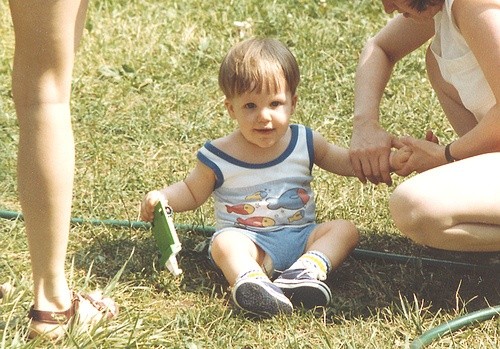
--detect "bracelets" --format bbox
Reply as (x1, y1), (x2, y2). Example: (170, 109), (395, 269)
(445, 142), (460, 163)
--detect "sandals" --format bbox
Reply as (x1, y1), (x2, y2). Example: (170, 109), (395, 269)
(25, 291), (119, 340)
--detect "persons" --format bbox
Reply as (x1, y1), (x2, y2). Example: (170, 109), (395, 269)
(140, 37), (440, 319)
(349, 0), (499, 312)
(0, 0), (120, 342)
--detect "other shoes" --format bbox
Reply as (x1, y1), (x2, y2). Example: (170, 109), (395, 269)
(273, 267), (332, 307)
(232, 278), (293, 317)
